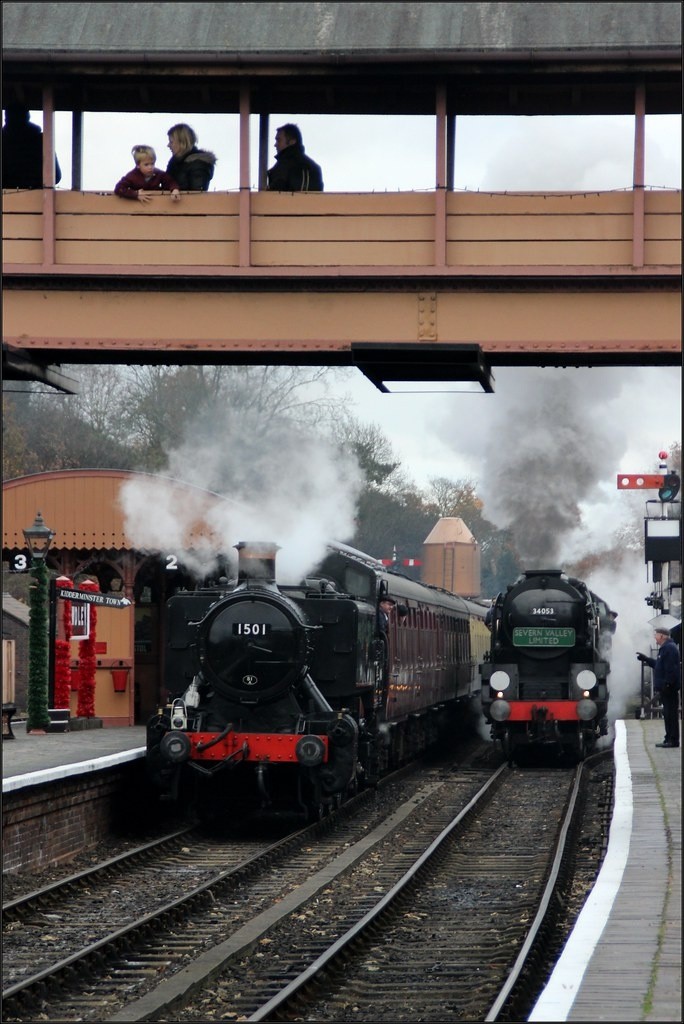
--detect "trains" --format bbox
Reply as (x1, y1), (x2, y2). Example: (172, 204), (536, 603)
(480, 569), (619, 768)
(134, 535), (492, 839)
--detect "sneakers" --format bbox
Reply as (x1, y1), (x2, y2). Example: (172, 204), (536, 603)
(654, 741), (680, 748)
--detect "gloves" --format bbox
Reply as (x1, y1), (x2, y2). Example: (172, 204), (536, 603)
(663, 683), (672, 697)
(635, 652), (647, 662)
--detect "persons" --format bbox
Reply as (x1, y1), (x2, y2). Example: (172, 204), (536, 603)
(636, 628), (682, 749)
(113, 146), (180, 204)
(1, 105), (61, 189)
(266, 123), (324, 192)
(377, 596), (396, 662)
(164, 123), (218, 192)
(396, 604), (409, 628)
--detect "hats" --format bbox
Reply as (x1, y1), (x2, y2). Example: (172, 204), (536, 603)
(654, 627), (670, 636)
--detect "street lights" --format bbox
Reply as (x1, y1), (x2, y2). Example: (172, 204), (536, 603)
(23, 511), (56, 735)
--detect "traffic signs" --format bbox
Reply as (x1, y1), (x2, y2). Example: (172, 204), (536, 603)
(59, 588), (132, 609)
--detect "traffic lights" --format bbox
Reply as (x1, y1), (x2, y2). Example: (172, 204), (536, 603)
(658, 470), (681, 504)
